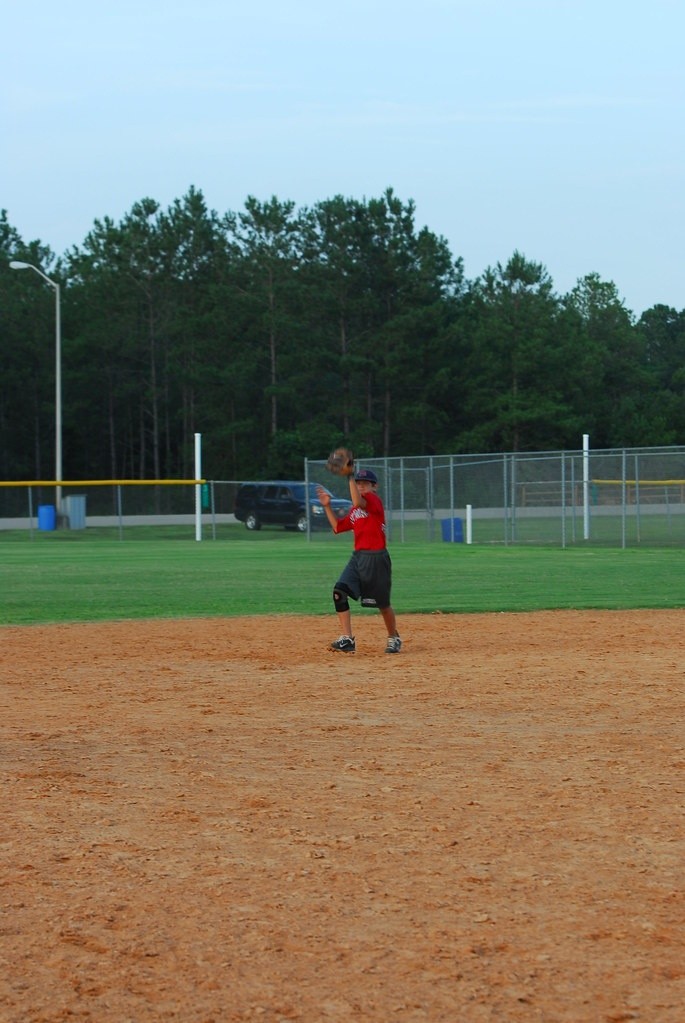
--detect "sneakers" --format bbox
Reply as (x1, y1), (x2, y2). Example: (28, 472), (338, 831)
(385, 630), (400, 652)
(326, 636), (356, 654)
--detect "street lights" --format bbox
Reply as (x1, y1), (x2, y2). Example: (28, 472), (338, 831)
(9, 261), (63, 519)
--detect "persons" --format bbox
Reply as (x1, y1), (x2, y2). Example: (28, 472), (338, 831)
(314, 446), (405, 654)
(281, 490), (291, 498)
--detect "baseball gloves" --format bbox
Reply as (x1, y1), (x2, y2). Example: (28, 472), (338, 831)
(325, 446), (355, 478)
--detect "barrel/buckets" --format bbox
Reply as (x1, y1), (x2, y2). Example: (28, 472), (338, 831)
(38, 505), (55, 530)
(441, 517), (463, 542)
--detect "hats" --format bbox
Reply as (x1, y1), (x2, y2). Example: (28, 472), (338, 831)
(353, 470), (377, 484)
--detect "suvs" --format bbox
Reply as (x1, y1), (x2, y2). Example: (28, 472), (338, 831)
(233, 481), (353, 533)
